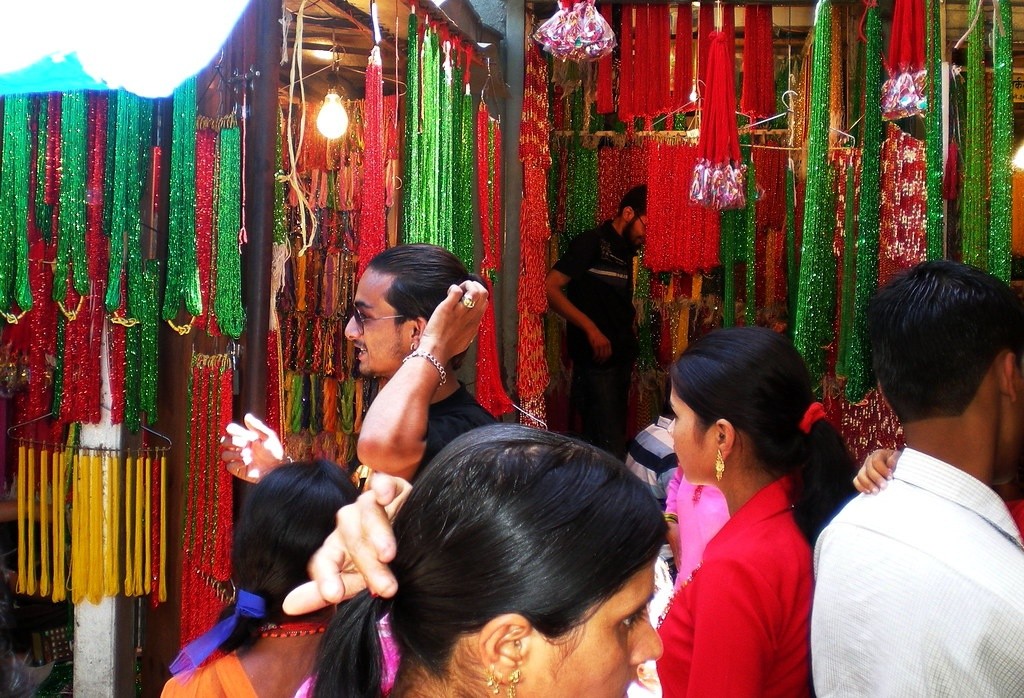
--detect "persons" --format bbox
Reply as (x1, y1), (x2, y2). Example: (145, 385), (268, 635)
(812, 260), (1024, 698)
(664, 463), (730, 593)
(623, 378), (679, 511)
(546, 184), (648, 464)
(655, 327), (860, 698)
(161, 459), (362, 698)
(853, 448), (1024, 537)
(219, 243), (498, 484)
(283, 423), (664, 698)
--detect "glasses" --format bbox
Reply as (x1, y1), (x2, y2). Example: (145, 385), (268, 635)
(638, 216), (646, 233)
(349, 303), (404, 335)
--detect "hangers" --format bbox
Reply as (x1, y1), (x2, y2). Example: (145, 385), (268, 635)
(511, 404), (547, 432)
(7, 397), (173, 454)
(190, 329), (236, 358)
(647, 79), (752, 132)
(480, 56), (504, 123)
(196, 49), (239, 117)
(278, 43), (407, 102)
(194, 565), (236, 605)
(739, 91), (856, 149)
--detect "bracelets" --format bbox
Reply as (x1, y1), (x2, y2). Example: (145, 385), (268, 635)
(286, 456), (292, 465)
(403, 352), (446, 388)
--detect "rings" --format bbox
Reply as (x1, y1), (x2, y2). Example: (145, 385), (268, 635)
(462, 295), (474, 308)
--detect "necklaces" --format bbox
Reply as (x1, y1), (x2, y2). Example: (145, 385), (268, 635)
(657, 563), (700, 627)
(263, 627), (325, 639)
